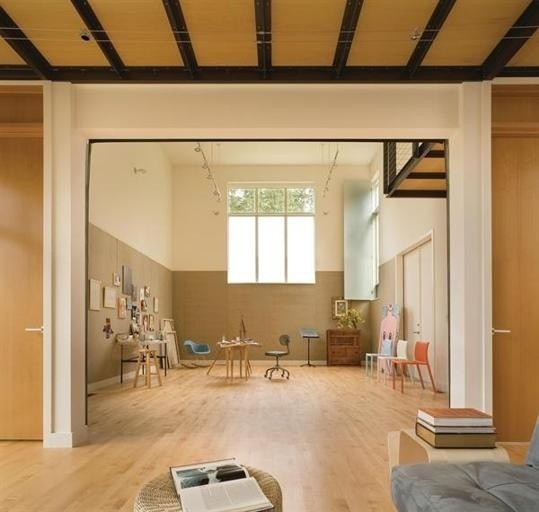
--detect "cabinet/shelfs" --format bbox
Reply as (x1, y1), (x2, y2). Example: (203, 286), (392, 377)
(326, 328), (364, 367)
(383, 140), (447, 200)
(397, 428), (511, 465)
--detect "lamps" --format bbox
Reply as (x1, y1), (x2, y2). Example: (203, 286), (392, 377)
(317, 142), (340, 198)
(195, 142), (224, 204)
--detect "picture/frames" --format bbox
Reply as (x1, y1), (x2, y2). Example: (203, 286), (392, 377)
(89, 266), (160, 332)
(164, 331), (180, 369)
(330, 295), (352, 321)
(161, 319), (175, 368)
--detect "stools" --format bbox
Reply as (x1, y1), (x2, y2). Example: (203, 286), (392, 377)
(133, 349), (163, 389)
(223, 345), (244, 380)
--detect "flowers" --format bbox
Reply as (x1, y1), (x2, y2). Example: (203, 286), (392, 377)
(336, 308), (366, 328)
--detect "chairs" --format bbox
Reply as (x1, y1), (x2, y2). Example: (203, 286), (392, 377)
(181, 339), (214, 372)
(263, 335), (292, 380)
(376, 340), (413, 386)
(391, 413), (539, 512)
(392, 341), (438, 394)
(366, 338), (394, 378)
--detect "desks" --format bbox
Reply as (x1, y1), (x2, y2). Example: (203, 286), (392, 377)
(118, 339), (169, 385)
(299, 329), (320, 367)
(206, 342), (259, 376)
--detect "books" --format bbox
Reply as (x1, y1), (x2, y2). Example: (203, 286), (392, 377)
(416, 407), (499, 449)
(169, 457), (275, 512)
